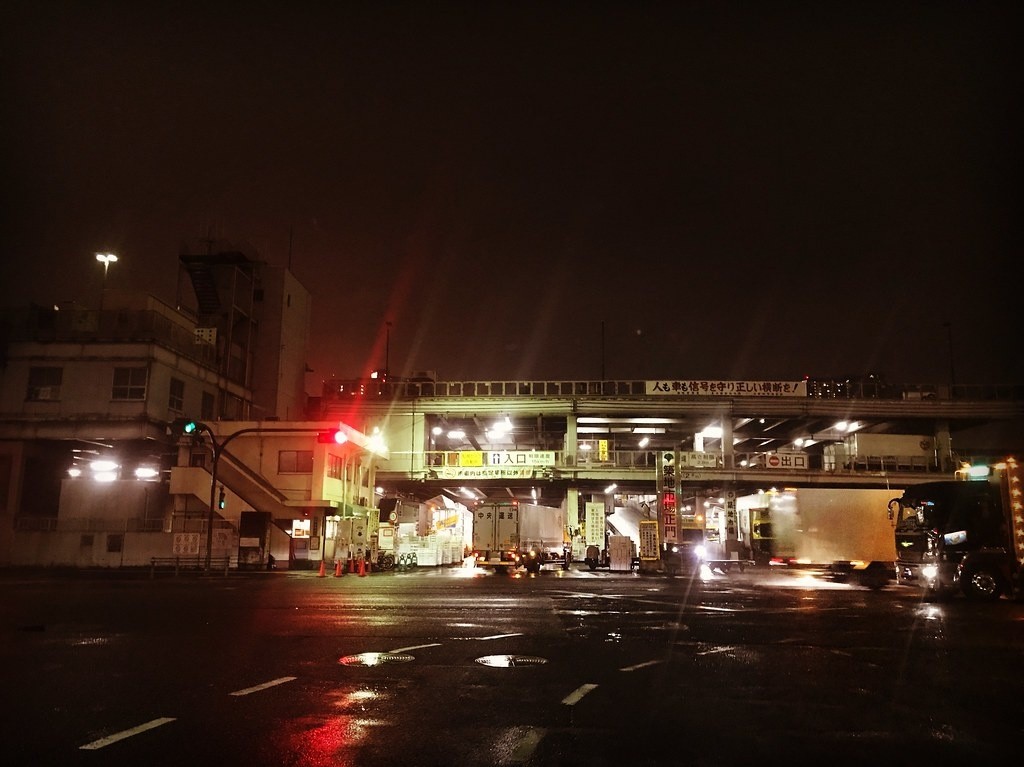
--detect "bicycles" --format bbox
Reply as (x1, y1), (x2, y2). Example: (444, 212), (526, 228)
(378, 550), (395, 572)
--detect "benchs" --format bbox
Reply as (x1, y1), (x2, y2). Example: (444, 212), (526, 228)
(149, 554), (230, 581)
(854, 453), (930, 473)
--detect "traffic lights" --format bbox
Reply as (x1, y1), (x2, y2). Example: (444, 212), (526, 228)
(219, 487), (226, 509)
(174, 421), (196, 432)
(316, 432), (347, 444)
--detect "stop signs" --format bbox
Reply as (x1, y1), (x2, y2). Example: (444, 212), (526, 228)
(769, 456), (780, 467)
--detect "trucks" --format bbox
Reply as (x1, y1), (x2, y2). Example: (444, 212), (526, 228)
(471, 498), (572, 574)
(886, 467), (1024, 601)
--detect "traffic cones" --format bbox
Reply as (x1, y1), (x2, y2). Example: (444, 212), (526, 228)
(348, 558), (356, 574)
(333, 559), (344, 577)
(358, 558), (372, 577)
(316, 560), (328, 578)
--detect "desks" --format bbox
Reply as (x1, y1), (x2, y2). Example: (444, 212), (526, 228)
(705, 559), (748, 574)
